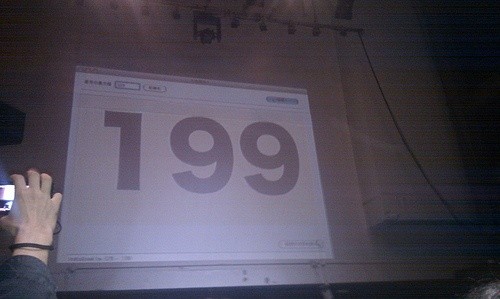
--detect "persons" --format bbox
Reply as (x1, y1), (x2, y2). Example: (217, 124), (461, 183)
(1, 169), (62, 299)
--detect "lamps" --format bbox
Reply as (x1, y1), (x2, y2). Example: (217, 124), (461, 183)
(69, 0), (365, 48)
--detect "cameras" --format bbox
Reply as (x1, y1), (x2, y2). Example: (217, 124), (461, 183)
(0, 183), (54, 216)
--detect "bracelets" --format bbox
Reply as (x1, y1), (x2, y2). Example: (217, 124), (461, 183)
(10, 243), (54, 250)
